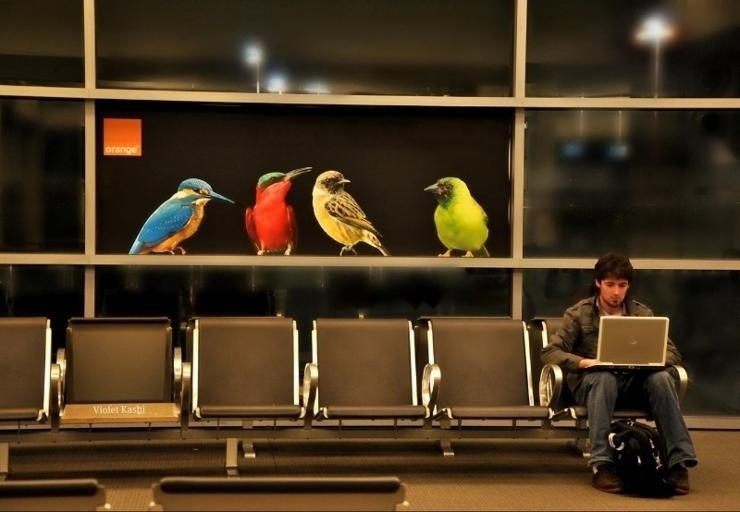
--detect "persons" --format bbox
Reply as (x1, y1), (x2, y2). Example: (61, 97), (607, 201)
(539, 252), (698, 496)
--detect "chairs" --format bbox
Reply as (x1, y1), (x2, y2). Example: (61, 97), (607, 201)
(310, 316), (441, 430)
(537, 315), (688, 425)
(422, 315), (562, 424)
(192, 315), (317, 431)
(49, 317), (187, 435)
(2, 316), (56, 434)
(148, 476), (411, 512)
(0, 474), (108, 511)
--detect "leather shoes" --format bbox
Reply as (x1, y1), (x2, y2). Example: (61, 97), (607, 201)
(591, 466), (621, 493)
(674, 470), (690, 495)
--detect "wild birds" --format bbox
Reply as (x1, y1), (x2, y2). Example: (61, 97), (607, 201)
(128, 176), (236, 256)
(423, 176), (494, 258)
(311, 170), (400, 257)
(243, 164), (314, 255)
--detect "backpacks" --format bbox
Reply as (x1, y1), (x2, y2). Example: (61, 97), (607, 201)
(608, 416), (669, 499)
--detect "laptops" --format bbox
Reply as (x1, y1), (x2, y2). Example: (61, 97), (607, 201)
(584, 316), (669, 373)
